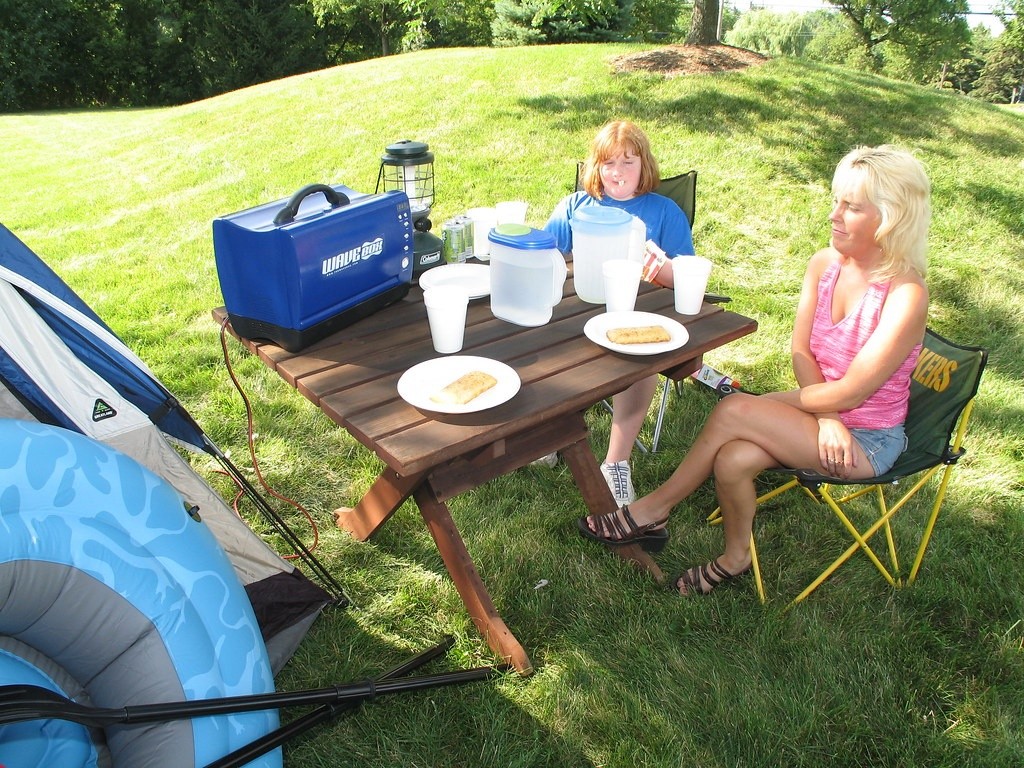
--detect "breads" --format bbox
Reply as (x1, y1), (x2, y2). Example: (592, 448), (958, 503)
(605, 325), (671, 344)
(439, 371), (497, 404)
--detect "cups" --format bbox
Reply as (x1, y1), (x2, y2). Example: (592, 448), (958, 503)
(466, 201), (528, 259)
(422, 289), (469, 354)
(602, 258), (642, 312)
(670, 255), (712, 315)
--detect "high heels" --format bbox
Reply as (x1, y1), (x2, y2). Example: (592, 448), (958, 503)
(578, 504), (669, 553)
(672, 554), (753, 598)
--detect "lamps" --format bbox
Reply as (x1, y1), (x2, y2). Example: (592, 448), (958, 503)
(378, 137), (447, 272)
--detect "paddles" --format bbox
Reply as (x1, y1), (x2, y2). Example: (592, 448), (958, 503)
(0, 663), (508, 733)
(203, 635), (456, 768)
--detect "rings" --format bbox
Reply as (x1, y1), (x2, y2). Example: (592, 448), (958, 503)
(835, 461), (844, 464)
(828, 458), (834, 463)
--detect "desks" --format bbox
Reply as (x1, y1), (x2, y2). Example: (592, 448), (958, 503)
(206, 244), (759, 677)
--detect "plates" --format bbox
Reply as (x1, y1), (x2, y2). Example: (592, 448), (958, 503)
(583, 311), (690, 356)
(418, 262), (490, 301)
(397, 356), (522, 415)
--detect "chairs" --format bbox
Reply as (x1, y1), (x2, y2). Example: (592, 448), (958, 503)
(702, 326), (989, 622)
(570, 157), (711, 456)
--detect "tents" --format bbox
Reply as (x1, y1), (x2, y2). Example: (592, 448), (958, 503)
(0, 222), (349, 690)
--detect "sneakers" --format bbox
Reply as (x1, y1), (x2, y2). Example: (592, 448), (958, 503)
(529, 451), (557, 469)
(600, 459), (634, 509)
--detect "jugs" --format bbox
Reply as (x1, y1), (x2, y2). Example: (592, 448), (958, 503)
(568, 205), (646, 305)
(487, 223), (567, 328)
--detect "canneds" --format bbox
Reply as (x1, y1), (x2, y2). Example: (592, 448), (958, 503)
(440, 215), (475, 264)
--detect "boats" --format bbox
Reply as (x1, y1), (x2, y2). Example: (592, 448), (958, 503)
(0, 419), (282, 768)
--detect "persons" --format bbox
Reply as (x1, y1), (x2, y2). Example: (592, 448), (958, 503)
(578, 144), (931, 596)
(528, 119), (696, 511)
